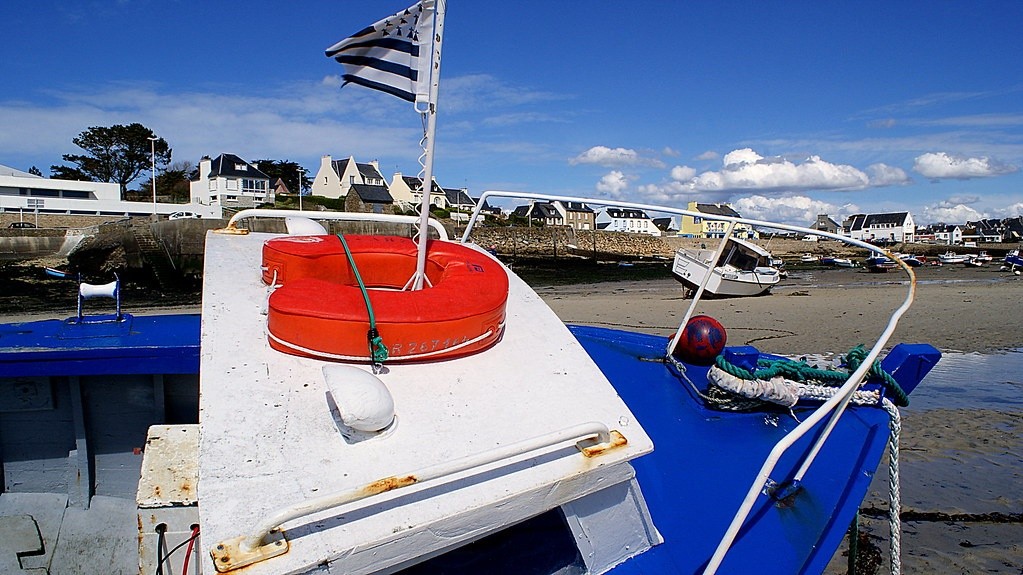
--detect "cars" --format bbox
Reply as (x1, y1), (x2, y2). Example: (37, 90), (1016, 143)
(168, 211), (202, 220)
(8, 222), (43, 228)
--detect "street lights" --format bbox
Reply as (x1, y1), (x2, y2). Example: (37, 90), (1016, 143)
(296, 169), (304, 211)
(458, 189), (465, 227)
(253, 179), (260, 219)
(596, 206), (601, 230)
(147, 137), (159, 214)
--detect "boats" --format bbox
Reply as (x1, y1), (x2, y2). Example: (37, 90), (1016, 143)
(832, 259), (860, 267)
(1005, 249), (1023, 267)
(865, 247), (890, 272)
(671, 233), (781, 298)
(938, 251), (965, 264)
(963, 251), (993, 267)
(44, 265), (78, 280)
(823, 255), (835, 264)
(800, 253), (822, 262)
(0, 1), (942, 575)
(902, 254), (927, 267)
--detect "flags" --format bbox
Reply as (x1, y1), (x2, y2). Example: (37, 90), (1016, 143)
(325, 0), (436, 104)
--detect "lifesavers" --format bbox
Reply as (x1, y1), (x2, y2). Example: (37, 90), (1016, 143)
(261, 234), (509, 364)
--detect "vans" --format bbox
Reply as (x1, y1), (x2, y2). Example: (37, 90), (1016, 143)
(802, 235), (817, 241)
